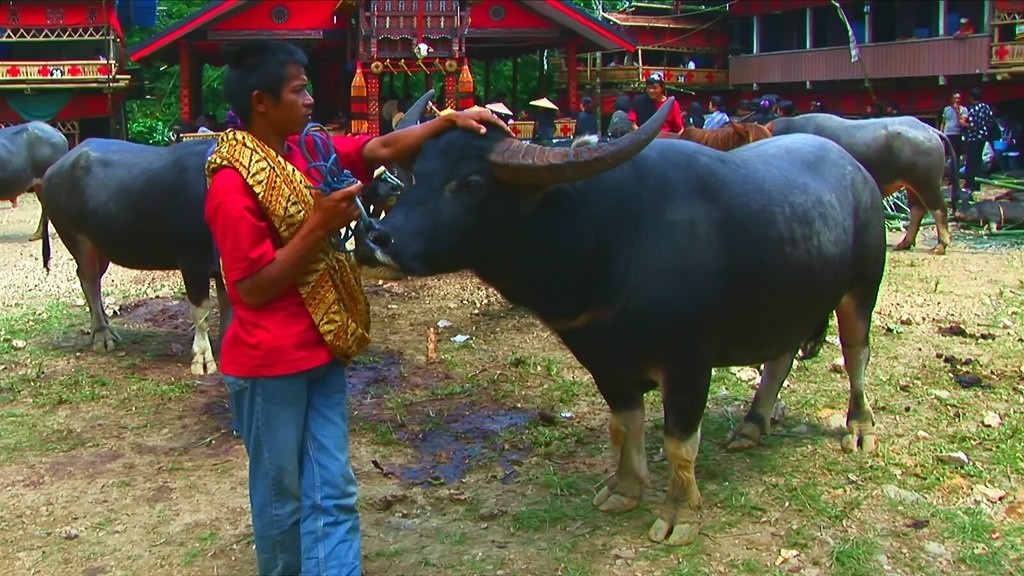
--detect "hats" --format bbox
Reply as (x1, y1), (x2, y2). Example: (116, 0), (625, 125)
(529, 98), (560, 111)
(645, 71), (663, 83)
(485, 102), (513, 116)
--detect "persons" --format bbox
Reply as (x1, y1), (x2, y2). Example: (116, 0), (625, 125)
(678, 53), (696, 83)
(953, 17), (976, 38)
(627, 71), (685, 139)
(487, 94), (905, 148)
(196, 113), (219, 133)
(939, 86), (1023, 195)
(609, 55), (623, 68)
(203, 41), (519, 576)
